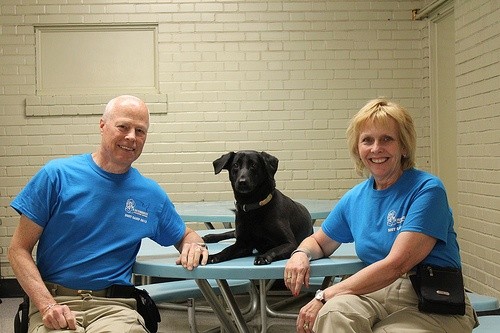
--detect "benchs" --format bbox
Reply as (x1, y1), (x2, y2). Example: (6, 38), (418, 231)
(136, 277), (347, 332)
(466, 292), (500, 333)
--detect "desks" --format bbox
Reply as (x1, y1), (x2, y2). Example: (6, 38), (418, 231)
(133, 228), (366, 333)
(171, 198), (341, 230)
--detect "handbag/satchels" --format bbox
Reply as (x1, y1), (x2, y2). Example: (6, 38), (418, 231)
(409, 264), (467, 317)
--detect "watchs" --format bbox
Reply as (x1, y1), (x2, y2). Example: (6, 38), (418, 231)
(315, 289), (326, 305)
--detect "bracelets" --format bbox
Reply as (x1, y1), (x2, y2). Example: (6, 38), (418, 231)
(290, 248), (312, 261)
(196, 243), (208, 250)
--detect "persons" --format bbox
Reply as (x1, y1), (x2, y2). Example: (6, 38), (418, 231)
(7, 95), (209, 333)
(283, 99), (477, 333)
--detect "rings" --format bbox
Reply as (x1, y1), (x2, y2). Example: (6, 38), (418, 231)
(286, 276), (292, 278)
(303, 324), (308, 328)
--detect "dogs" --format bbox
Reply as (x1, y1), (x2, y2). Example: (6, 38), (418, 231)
(202, 150), (317, 266)
